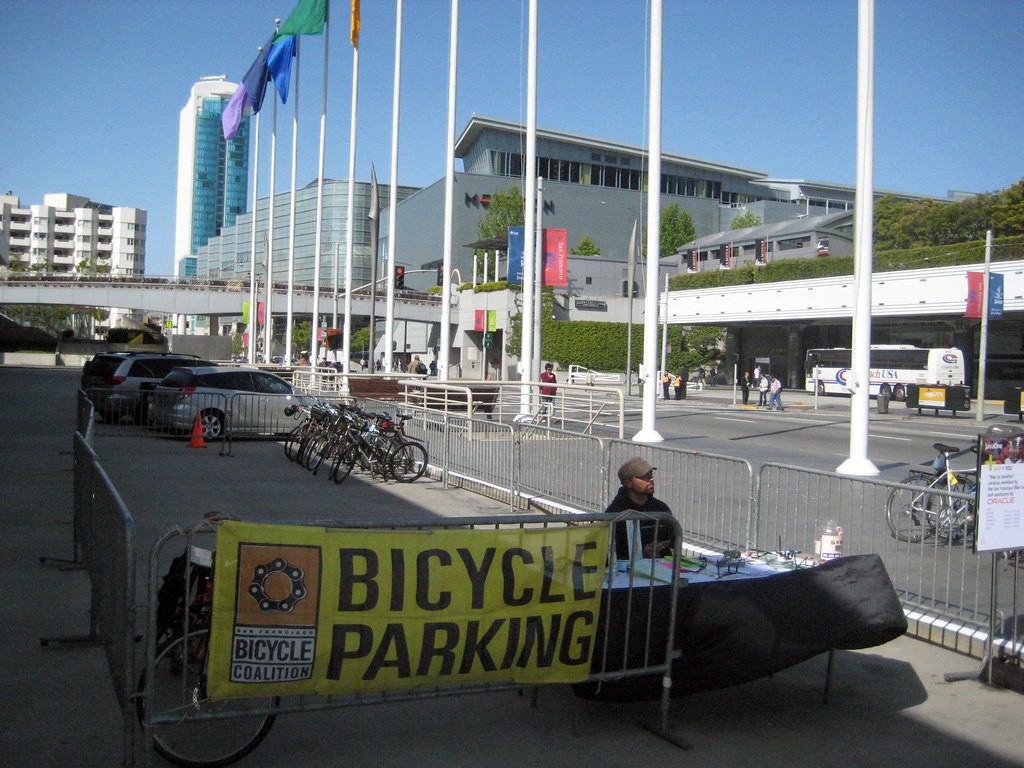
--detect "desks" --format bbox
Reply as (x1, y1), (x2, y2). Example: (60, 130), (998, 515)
(572, 551), (907, 706)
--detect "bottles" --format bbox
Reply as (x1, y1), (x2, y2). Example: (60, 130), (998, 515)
(821, 519), (841, 565)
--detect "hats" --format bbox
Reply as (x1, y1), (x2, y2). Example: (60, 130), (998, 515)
(618, 457), (658, 482)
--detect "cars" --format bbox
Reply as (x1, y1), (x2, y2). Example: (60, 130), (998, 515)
(233, 356), (248, 363)
(272, 356), (283, 363)
(148, 365), (315, 441)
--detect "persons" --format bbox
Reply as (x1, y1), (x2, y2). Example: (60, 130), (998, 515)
(996, 440), (1024, 464)
(698, 364), (718, 387)
(483, 375), (496, 420)
(408, 355), (422, 374)
(539, 363), (560, 424)
(604, 457), (676, 560)
(429, 360), (436, 376)
(663, 370), (671, 400)
(674, 373), (682, 400)
(740, 365), (784, 410)
(360, 357), (404, 372)
(300, 355), (344, 388)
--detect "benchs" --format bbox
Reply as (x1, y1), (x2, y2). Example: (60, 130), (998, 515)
(347, 378), (499, 421)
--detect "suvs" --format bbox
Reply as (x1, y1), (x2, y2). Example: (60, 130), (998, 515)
(80, 351), (220, 425)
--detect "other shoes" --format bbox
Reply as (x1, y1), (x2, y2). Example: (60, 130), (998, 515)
(542, 418), (546, 423)
(553, 419), (559, 424)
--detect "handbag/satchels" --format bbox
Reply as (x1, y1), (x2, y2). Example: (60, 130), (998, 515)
(415, 361), (428, 374)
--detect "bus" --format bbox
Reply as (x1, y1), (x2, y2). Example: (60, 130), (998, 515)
(806, 345), (965, 402)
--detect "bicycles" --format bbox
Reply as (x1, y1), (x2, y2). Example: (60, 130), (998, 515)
(284, 391), (428, 487)
(886, 434), (1024, 559)
(141, 513), (281, 768)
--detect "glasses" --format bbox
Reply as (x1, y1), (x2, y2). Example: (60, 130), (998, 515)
(547, 369), (552, 371)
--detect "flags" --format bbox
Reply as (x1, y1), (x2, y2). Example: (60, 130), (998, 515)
(221, 0), (331, 139)
(351, 0), (361, 48)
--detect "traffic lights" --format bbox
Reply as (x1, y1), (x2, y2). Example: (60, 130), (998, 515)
(395, 266), (405, 289)
(437, 264), (443, 285)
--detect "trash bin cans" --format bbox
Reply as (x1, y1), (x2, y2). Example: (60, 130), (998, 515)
(300, 351), (309, 366)
(878, 393), (890, 413)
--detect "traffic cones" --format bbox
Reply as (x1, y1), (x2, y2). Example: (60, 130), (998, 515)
(188, 411), (207, 448)
